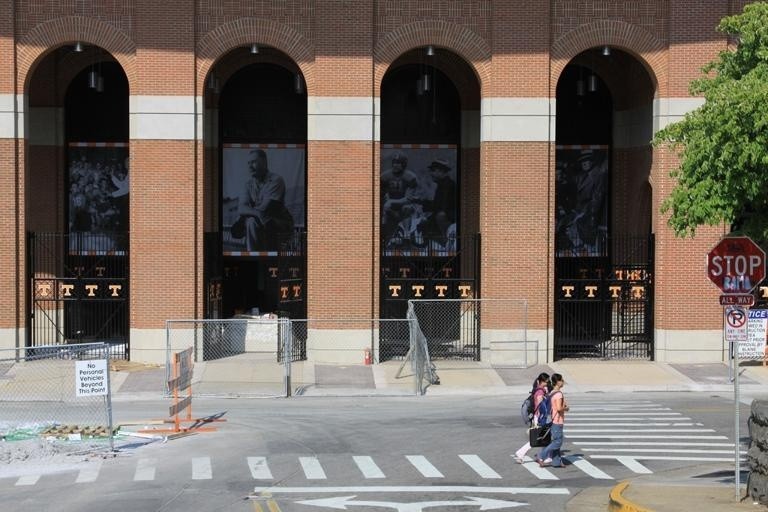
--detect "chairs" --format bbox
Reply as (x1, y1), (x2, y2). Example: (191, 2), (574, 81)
(514, 453), (567, 468)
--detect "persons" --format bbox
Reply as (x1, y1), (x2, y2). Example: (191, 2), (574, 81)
(512, 373), (554, 464)
(68, 152), (129, 252)
(554, 159), (578, 251)
(406, 159), (457, 247)
(534, 373), (569, 469)
(567, 147), (605, 250)
(230, 150), (296, 253)
(380, 151), (420, 251)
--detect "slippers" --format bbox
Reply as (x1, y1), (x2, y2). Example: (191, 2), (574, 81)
(537, 390), (561, 427)
(520, 388), (545, 421)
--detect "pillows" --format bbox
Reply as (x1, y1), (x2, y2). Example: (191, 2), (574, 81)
(391, 150), (408, 167)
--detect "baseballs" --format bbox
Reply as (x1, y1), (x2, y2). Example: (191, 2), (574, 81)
(365, 348), (371, 364)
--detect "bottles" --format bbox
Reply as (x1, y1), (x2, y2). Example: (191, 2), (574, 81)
(427, 159), (452, 171)
(577, 150), (594, 164)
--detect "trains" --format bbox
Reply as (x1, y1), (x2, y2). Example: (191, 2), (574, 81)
(707, 235), (766, 295)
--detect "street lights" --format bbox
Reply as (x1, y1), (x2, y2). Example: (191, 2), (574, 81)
(529, 426), (552, 448)
